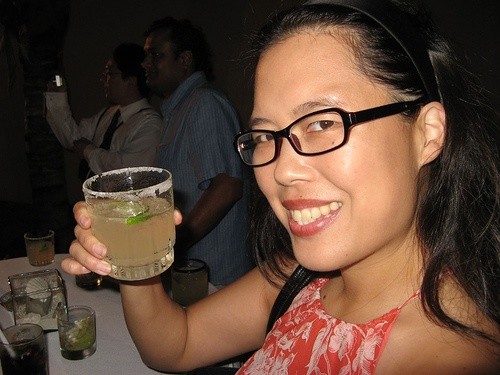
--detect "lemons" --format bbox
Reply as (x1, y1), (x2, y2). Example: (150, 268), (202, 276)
(113, 201), (150, 225)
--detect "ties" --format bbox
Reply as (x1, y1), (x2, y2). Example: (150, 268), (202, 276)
(88, 109), (121, 180)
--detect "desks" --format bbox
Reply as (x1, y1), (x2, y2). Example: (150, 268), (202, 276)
(0, 254), (241, 375)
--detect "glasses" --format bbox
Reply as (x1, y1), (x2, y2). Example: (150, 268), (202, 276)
(232, 97), (422, 168)
(97, 67), (124, 78)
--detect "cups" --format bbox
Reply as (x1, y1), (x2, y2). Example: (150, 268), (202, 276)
(0, 287), (25, 313)
(82, 166), (176, 281)
(0, 323), (46, 375)
(8, 269), (69, 333)
(169, 258), (209, 308)
(24, 229), (54, 267)
(57, 305), (97, 361)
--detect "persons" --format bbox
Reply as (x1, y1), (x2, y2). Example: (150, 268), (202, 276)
(48, 42), (164, 176)
(60, 0), (500, 375)
(141, 18), (249, 290)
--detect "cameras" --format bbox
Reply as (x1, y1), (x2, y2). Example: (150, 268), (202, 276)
(51, 70), (63, 88)
